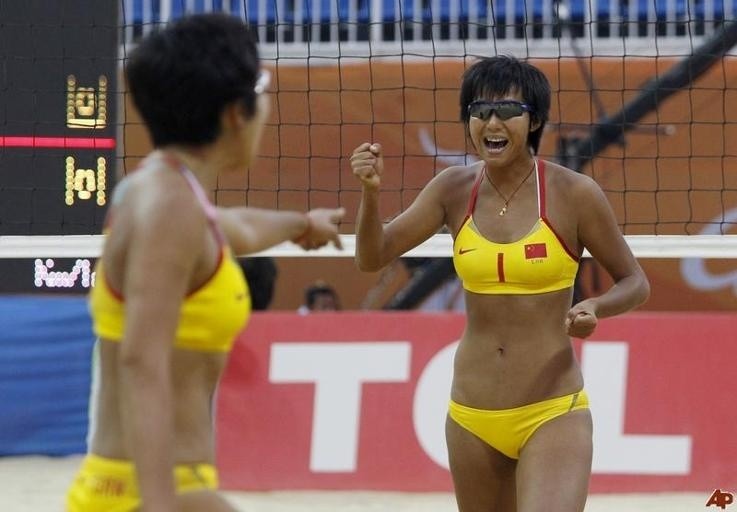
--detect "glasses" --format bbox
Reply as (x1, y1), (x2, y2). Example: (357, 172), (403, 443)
(467, 99), (533, 122)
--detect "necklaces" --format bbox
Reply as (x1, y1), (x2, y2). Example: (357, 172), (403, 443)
(483, 162), (536, 216)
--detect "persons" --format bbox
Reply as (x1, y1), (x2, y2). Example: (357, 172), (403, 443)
(65, 10), (348, 511)
(304, 280), (338, 312)
(349, 52), (651, 510)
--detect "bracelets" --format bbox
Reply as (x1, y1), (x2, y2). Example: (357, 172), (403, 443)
(291, 213), (313, 245)
